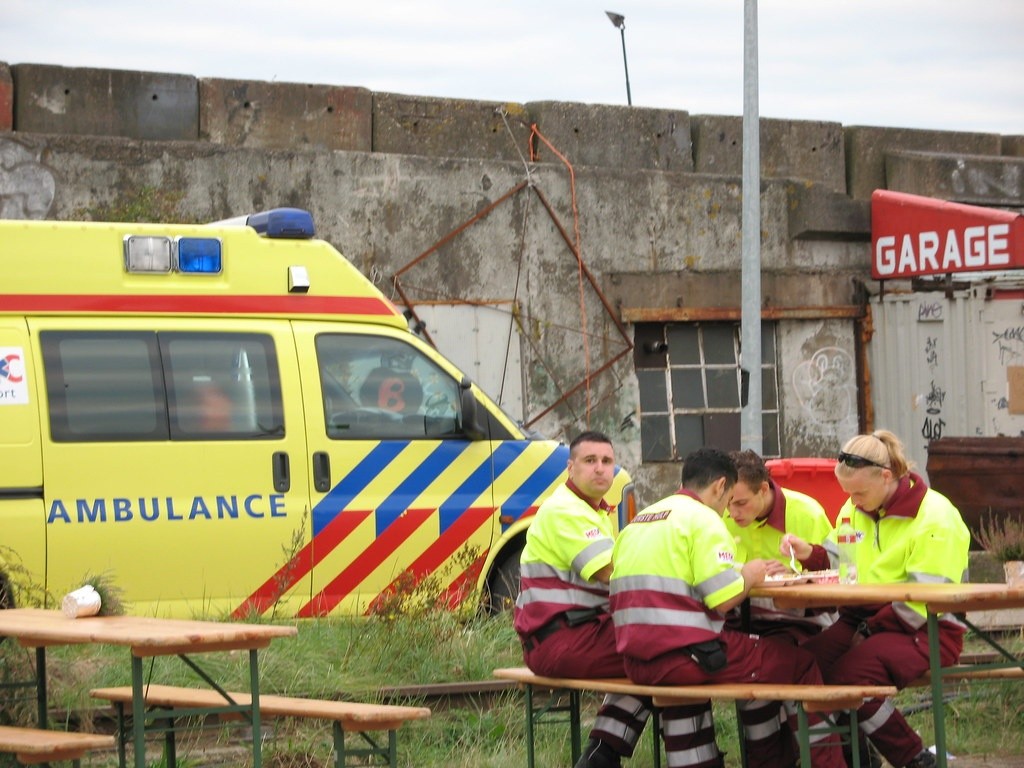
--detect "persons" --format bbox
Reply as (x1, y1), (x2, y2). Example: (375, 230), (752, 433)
(512, 429), (971, 768)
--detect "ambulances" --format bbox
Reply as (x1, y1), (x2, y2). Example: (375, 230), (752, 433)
(0, 207), (647, 620)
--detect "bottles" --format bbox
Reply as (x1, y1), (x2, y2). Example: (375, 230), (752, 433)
(838, 517), (859, 584)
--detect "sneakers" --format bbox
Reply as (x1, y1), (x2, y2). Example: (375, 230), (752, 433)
(574, 740), (623, 768)
(906, 748), (937, 768)
(847, 742), (882, 768)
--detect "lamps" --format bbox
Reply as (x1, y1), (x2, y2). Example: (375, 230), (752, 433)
(606, 12), (631, 106)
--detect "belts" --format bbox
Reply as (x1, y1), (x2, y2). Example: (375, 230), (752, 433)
(525, 618), (561, 653)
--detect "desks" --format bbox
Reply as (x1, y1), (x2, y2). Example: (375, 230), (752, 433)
(0, 609), (297, 768)
(739, 583), (1024, 768)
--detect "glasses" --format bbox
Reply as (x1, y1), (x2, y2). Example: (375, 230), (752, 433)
(838, 451), (890, 470)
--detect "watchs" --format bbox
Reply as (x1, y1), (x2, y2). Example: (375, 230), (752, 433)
(857, 618), (870, 639)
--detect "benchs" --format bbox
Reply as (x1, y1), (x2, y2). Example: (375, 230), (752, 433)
(0, 726), (116, 768)
(909, 667), (1024, 687)
(493, 667), (898, 768)
(90, 687), (431, 768)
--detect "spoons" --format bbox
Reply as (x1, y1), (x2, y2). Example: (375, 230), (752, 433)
(787, 535), (801, 575)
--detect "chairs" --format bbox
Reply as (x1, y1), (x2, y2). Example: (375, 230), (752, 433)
(266, 353), (359, 428)
(184, 383), (233, 434)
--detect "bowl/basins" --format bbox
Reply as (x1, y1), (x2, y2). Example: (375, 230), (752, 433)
(757, 571), (838, 587)
